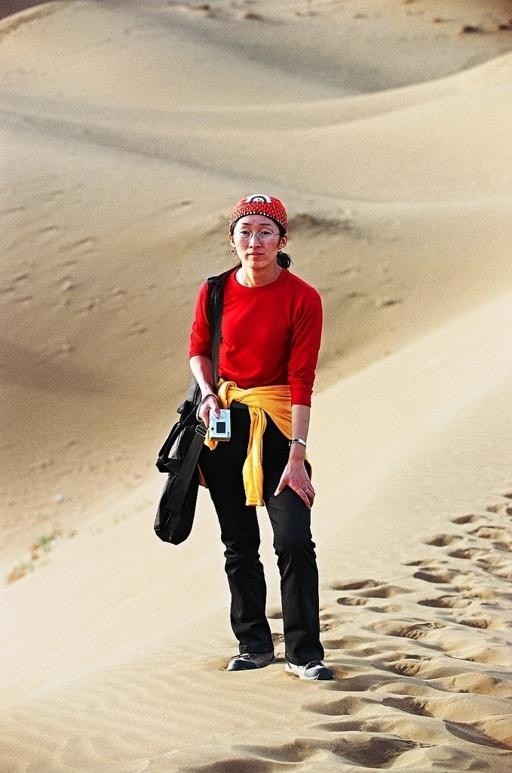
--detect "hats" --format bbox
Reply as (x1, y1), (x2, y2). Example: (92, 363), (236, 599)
(229, 193), (288, 236)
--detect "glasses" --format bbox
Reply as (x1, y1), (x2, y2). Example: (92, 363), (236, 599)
(238, 230), (281, 241)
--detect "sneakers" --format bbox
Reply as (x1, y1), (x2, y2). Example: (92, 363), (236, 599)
(285, 660), (335, 680)
(228, 652), (276, 671)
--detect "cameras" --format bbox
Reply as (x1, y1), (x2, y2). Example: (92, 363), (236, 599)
(209, 409), (231, 441)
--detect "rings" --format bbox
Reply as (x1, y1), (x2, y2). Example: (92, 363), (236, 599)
(305, 489), (309, 493)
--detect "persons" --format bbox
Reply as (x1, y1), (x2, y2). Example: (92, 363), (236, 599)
(187, 193), (339, 684)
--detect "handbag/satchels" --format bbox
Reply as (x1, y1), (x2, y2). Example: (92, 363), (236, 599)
(154, 400), (207, 545)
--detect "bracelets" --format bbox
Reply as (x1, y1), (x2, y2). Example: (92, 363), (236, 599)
(289, 437), (308, 448)
